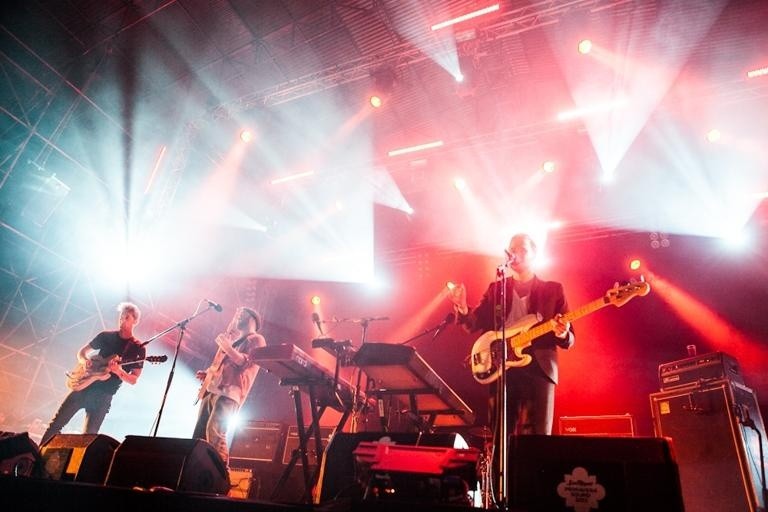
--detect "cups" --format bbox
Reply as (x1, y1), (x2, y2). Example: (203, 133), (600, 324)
(686, 344), (696, 357)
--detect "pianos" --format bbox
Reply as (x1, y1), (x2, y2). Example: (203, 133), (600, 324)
(353, 341), (476, 427)
(249, 344), (375, 413)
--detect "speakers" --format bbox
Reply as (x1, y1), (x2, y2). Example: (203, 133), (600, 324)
(280, 425), (338, 466)
(558, 414), (637, 437)
(103, 435), (232, 495)
(36, 433), (121, 482)
(648, 378), (768, 512)
(318, 431), (484, 507)
(504, 434), (686, 512)
(227, 421), (287, 464)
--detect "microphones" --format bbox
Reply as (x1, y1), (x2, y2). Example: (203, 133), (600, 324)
(205, 298), (223, 312)
(311, 312), (324, 336)
(505, 248), (521, 265)
(432, 312), (456, 338)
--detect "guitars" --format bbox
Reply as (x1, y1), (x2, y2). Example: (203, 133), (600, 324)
(66, 356), (168, 392)
(194, 308), (245, 399)
(471, 276), (649, 384)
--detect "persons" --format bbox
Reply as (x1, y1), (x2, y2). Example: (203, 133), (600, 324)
(190, 306), (267, 470)
(37, 301), (146, 446)
(448, 231), (575, 438)
